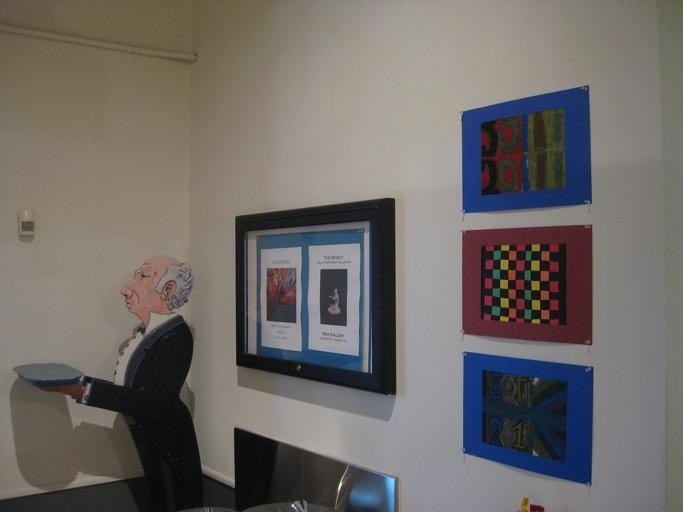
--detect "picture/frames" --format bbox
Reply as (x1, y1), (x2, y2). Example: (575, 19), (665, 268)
(462, 350), (591, 484)
(237, 196), (396, 397)
(461, 226), (593, 345)
(463, 90), (592, 213)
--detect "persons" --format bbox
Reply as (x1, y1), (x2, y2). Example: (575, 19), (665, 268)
(38, 256), (203, 512)
